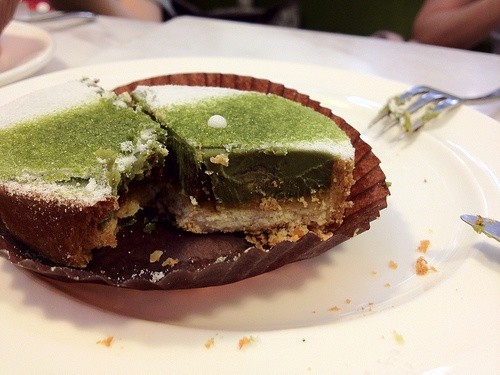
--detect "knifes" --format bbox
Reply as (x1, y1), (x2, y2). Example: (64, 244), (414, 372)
(460, 215), (500, 243)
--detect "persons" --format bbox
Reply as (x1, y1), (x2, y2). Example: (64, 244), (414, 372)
(52, 0), (175, 22)
(413, 0), (500, 51)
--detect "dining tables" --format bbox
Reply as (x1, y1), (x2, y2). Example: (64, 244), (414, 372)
(0, 10), (500, 375)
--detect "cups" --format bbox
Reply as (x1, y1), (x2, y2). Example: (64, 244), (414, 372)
(1, 0), (22, 34)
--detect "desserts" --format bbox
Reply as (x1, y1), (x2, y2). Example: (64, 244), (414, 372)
(130, 83), (357, 236)
(0, 76), (170, 269)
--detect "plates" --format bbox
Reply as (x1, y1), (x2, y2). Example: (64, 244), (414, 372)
(0, 58), (500, 375)
(0, 20), (54, 87)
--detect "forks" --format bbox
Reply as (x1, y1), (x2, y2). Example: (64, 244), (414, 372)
(367, 88), (500, 143)
